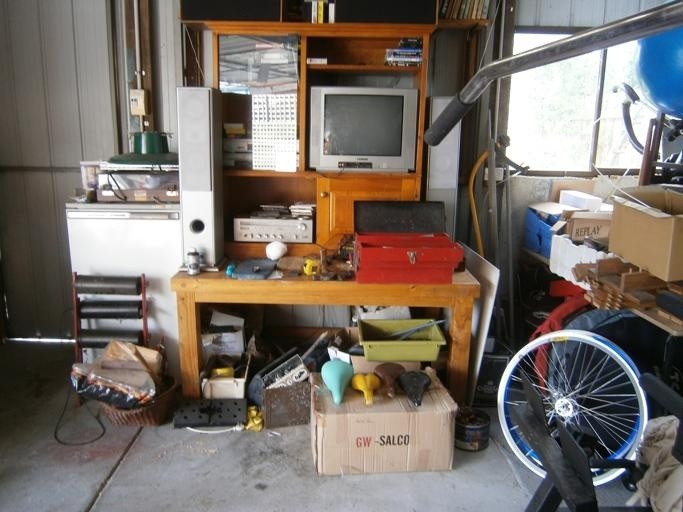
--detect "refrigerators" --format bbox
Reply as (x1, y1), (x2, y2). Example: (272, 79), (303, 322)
(66, 203), (183, 380)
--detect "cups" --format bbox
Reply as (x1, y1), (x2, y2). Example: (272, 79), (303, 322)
(303, 252), (320, 277)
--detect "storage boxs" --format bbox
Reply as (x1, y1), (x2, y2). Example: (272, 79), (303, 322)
(524, 190), (617, 290)
(608, 185), (682, 282)
(310, 368), (457, 471)
(327, 315), (446, 373)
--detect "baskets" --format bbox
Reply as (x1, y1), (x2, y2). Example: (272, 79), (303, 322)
(99, 377), (177, 427)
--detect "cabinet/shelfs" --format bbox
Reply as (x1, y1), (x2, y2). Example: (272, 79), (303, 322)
(171, 22), (435, 255)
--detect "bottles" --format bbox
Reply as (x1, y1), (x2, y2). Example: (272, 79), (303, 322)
(186, 252), (200, 277)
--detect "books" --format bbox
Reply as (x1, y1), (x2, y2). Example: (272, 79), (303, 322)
(384, 47), (422, 67)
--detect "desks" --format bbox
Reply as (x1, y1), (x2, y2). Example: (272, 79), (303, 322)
(171, 260), (483, 398)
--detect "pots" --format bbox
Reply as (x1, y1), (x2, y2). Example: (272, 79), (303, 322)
(128, 131), (175, 154)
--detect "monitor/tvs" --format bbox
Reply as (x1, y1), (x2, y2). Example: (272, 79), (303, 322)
(308, 85), (419, 173)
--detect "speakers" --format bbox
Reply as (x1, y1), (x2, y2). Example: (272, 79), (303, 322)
(176, 87), (228, 273)
(425, 96), (462, 243)
